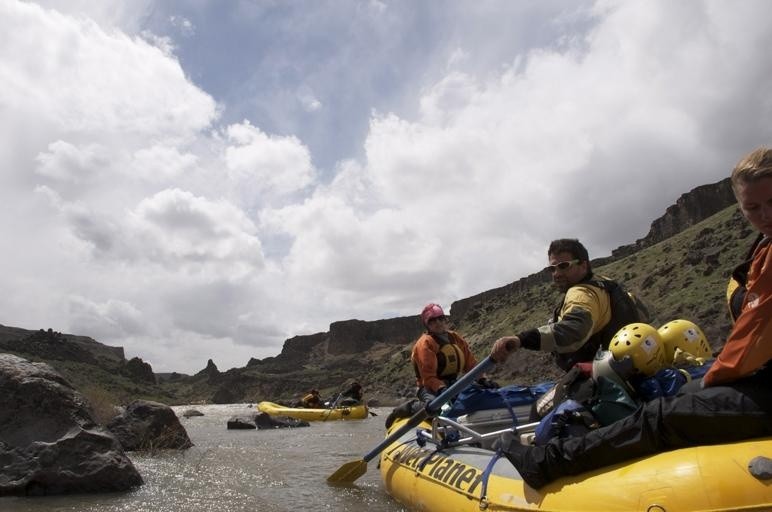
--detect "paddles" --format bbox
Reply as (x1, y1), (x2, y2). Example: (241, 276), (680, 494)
(327, 340), (517, 484)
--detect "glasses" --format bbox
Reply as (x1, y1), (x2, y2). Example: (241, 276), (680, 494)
(428, 315), (446, 325)
(548, 259), (580, 272)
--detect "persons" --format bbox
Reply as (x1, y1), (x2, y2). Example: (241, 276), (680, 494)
(385, 303), (502, 429)
(297, 389), (321, 409)
(489, 236), (649, 378)
(339, 381), (363, 408)
(501, 144), (771, 492)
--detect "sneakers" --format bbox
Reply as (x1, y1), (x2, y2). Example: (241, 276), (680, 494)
(497, 428), (554, 491)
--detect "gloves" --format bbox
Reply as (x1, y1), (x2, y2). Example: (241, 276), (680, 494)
(479, 378), (499, 388)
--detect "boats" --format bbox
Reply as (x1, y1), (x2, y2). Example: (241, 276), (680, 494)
(257, 400), (369, 421)
(380, 411), (772, 512)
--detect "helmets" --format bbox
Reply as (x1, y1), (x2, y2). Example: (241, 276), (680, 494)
(658, 319), (713, 368)
(422, 304), (443, 325)
(592, 350), (638, 398)
(611, 322), (666, 378)
